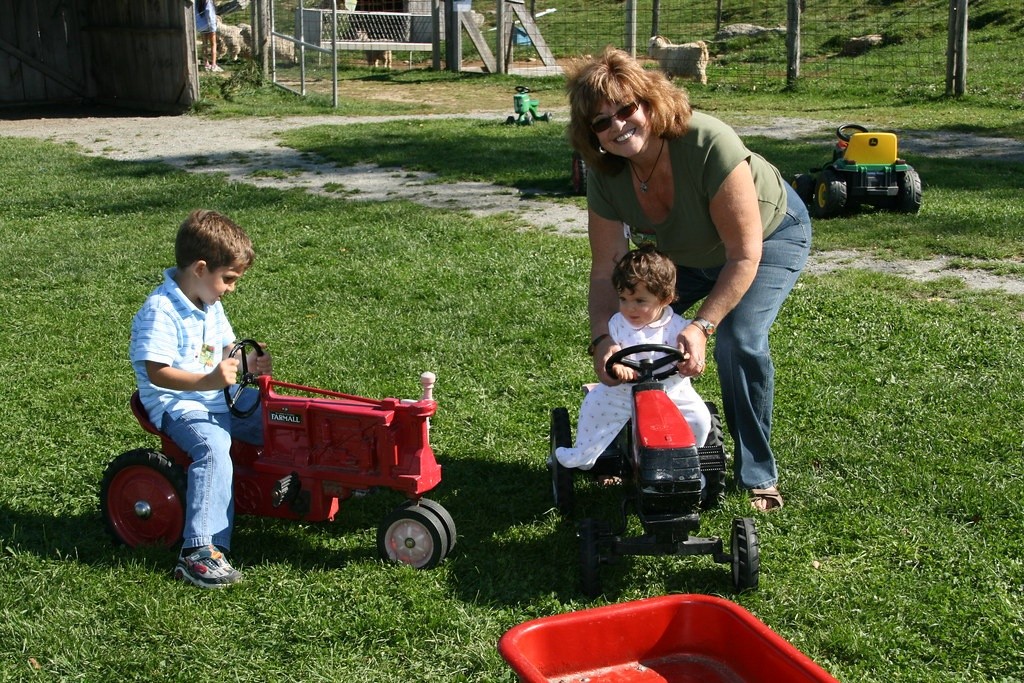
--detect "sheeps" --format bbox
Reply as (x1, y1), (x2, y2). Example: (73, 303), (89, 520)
(215, 15), (298, 65)
(648, 35), (710, 85)
(356, 30), (395, 69)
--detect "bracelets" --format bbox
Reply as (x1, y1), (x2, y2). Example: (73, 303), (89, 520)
(587, 333), (608, 356)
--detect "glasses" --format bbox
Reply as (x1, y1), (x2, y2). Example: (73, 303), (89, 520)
(589, 96), (642, 134)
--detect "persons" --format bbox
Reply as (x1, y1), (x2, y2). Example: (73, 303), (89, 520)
(130, 209), (274, 588)
(561, 48), (813, 513)
(195, 0), (224, 72)
(556, 248), (712, 470)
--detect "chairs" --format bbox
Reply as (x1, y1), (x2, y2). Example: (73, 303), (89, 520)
(130, 388), (173, 444)
(844, 131), (897, 164)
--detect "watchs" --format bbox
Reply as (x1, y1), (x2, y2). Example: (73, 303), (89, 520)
(694, 317), (714, 336)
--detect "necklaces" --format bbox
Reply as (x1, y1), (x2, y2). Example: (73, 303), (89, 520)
(630, 139), (665, 192)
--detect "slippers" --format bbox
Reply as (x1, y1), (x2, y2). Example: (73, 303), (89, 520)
(600, 475), (622, 486)
(745, 486), (783, 511)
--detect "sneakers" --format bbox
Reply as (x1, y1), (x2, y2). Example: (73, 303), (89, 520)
(174, 544), (243, 588)
